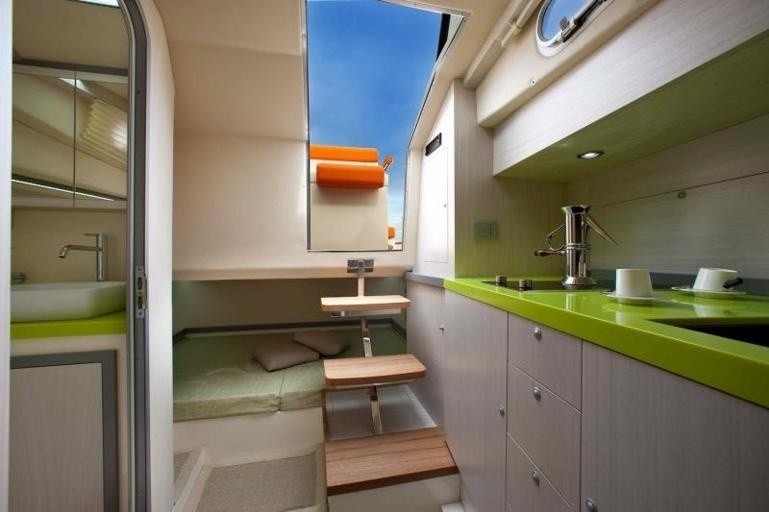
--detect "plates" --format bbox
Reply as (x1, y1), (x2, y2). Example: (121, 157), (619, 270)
(599, 291), (670, 306)
(670, 286), (751, 299)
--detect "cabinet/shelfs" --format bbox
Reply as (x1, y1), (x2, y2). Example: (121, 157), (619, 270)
(404, 270), (768, 511)
(411, 78), (568, 278)
(11, 311), (130, 511)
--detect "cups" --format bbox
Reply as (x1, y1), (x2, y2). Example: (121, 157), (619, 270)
(692, 266), (744, 290)
(613, 266), (657, 297)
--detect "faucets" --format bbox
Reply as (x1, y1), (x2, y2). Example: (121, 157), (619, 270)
(533, 205), (620, 286)
(59, 232), (108, 281)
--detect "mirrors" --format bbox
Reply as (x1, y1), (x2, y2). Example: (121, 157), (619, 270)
(13, 56), (129, 213)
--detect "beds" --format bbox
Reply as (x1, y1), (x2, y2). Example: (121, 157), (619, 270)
(173, 326), (411, 471)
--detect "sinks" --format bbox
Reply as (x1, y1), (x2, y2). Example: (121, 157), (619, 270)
(642, 317), (769, 347)
(11, 281), (127, 323)
